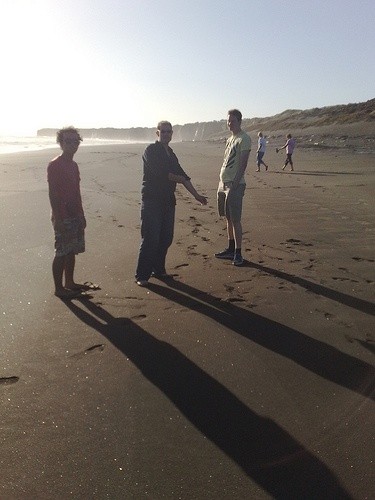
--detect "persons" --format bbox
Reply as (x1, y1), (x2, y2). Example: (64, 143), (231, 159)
(214, 109), (253, 265)
(46, 125), (90, 299)
(256, 132), (268, 172)
(135, 121), (209, 286)
(276, 134), (294, 171)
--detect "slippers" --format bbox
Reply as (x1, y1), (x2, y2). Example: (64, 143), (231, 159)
(77, 289), (90, 296)
(84, 281), (99, 289)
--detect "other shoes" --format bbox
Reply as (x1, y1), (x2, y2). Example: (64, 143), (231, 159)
(152, 269), (165, 276)
(233, 253), (244, 265)
(137, 280), (149, 286)
(214, 248), (234, 259)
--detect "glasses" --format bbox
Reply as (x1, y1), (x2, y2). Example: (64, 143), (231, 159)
(64, 139), (79, 144)
(158, 129), (172, 133)
